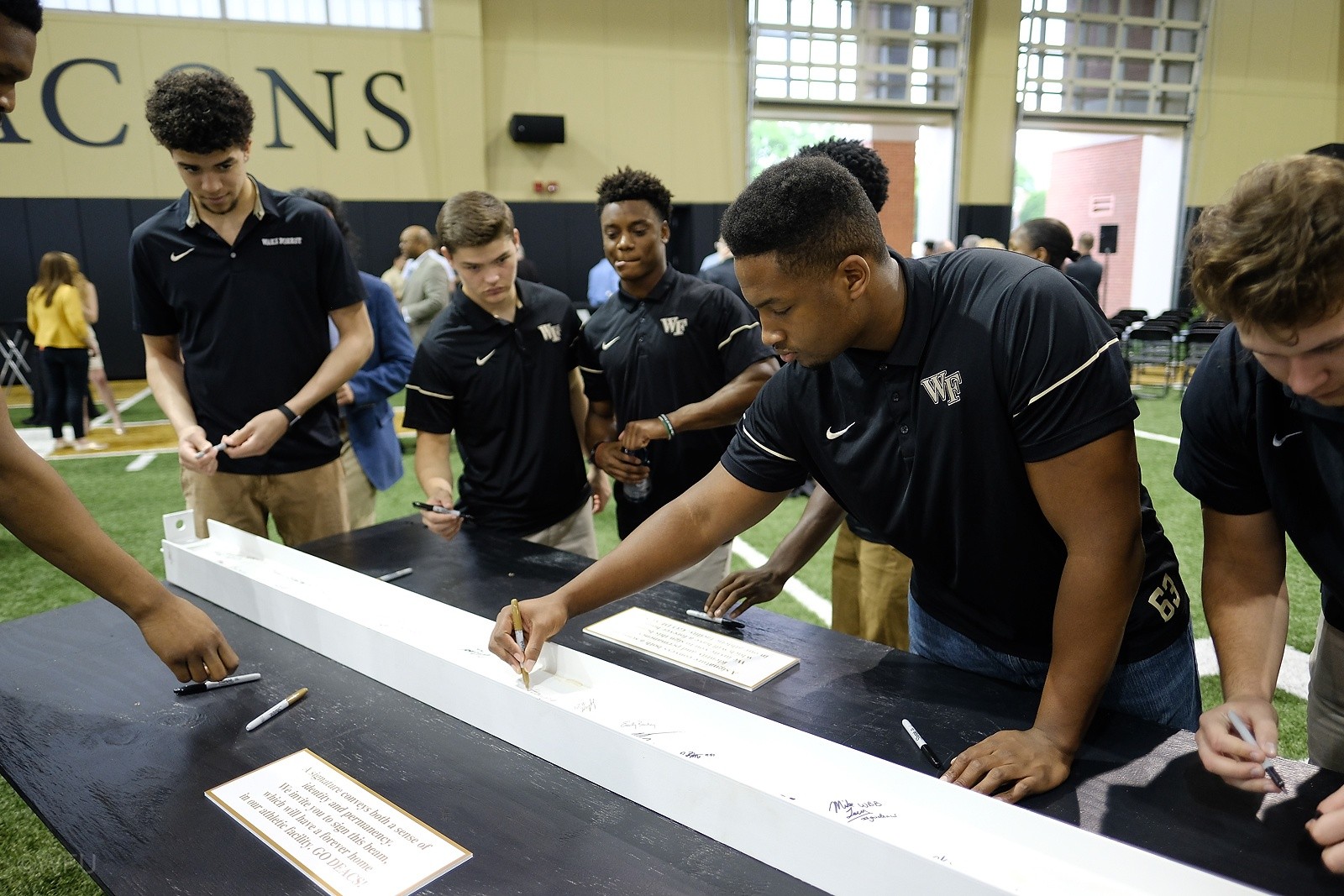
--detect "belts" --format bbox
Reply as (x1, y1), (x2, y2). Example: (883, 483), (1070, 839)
(337, 418), (347, 432)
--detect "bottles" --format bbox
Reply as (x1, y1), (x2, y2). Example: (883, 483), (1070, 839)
(625, 446), (650, 505)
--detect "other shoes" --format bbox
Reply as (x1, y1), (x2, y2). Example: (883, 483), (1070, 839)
(57, 440), (73, 451)
(114, 425), (123, 435)
(75, 442), (105, 451)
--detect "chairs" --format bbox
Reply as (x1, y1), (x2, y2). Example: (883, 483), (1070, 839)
(1097, 307), (1234, 394)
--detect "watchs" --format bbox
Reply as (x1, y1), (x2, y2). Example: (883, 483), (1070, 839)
(277, 404), (302, 429)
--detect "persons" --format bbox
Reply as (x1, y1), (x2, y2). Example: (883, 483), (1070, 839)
(564, 168), (781, 594)
(587, 257), (621, 316)
(20, 252), (124, 451)
(401, 191), (611, 560)
(279, 189), (416, 531)
(131, 73), (375, 547)
(1173, 142), (1344, 873)
(697, 135), (1102, 647)
(487, 151), (1203, 805)
(380, 225), (543, 355)
(0, 0), (241, 683)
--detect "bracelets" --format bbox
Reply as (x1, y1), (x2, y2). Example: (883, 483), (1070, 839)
(658, 414), (676, 441)
(588, 440), (605, 469)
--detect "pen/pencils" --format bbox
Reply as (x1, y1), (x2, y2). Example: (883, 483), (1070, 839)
(510, 599), (529, 691)
(902, 719), (944, 771)
(173, 674), (260, 696)
(195, 443), (229, 459)
(1227, 709), (1288, 795)
(376, 567), (412, 582)
(686, 610), (745, 628)
(412, 502), (474, 519)
(246, 688), (308, 731)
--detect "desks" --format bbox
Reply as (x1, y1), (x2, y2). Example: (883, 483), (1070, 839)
(0, 513), (1344, 896)
